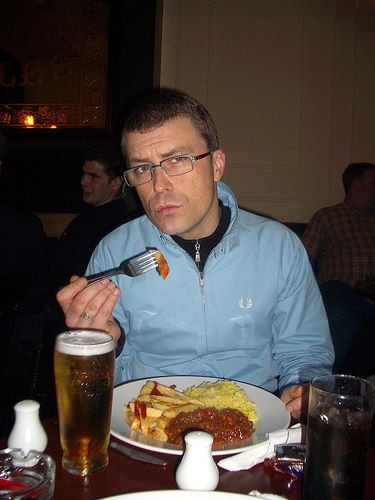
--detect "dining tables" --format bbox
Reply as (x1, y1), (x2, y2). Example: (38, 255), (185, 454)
(0, 416), (374, 500)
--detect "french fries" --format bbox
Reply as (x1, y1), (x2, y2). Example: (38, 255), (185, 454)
(127, 381), (202, 441)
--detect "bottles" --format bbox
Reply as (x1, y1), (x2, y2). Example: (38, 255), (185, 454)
(175, 430), (220, 491)
(9, 399), (48, 468)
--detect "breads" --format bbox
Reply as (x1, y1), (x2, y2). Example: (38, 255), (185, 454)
(149, 250), (169, 279)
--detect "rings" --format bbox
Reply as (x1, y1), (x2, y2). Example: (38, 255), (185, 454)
(80, 313), (95, 322)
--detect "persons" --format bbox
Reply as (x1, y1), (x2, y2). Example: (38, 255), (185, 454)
(57, 87), (338, 419)
(300, 161), (375, 380)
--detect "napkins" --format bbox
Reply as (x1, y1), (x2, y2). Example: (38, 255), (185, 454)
(217, 422), (307, 473)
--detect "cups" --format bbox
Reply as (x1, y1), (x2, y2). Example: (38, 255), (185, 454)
(303, 375), (375, 500)
(53, 328), (116, 476)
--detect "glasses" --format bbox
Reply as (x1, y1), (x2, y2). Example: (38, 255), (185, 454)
(123, 152), (211, 187)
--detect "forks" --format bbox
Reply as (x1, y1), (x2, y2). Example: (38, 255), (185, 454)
(78, 249), (162, 285)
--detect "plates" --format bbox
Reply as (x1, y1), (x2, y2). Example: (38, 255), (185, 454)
(109, 374), (291, 456)
(98, 489), (276, 500)
